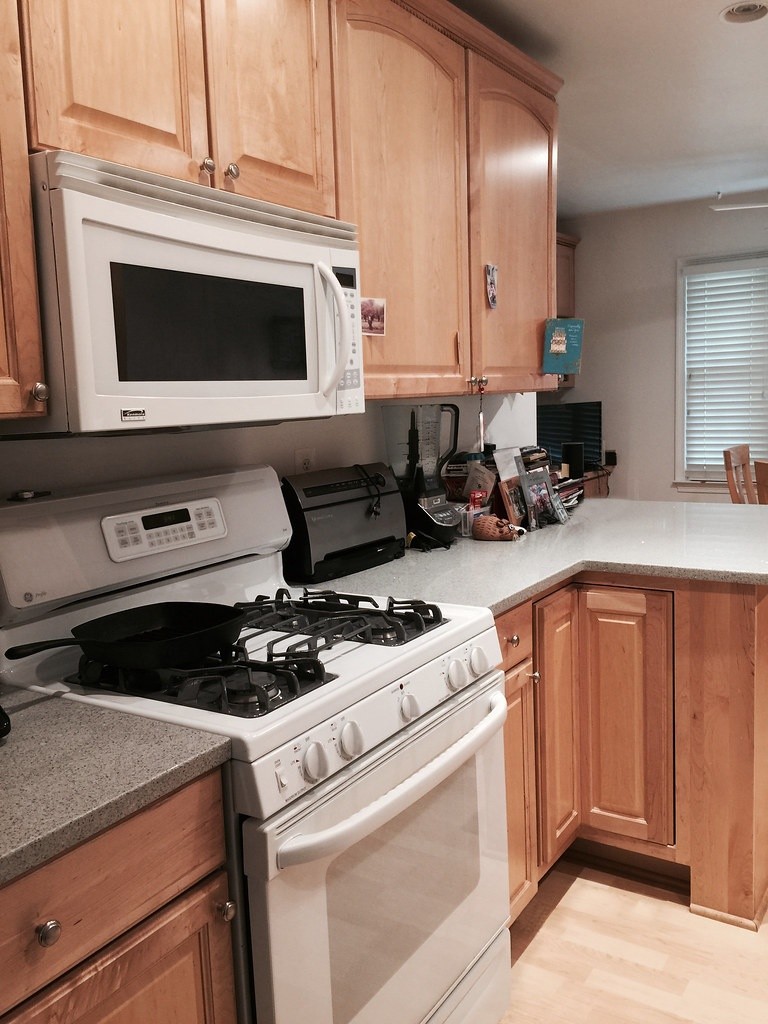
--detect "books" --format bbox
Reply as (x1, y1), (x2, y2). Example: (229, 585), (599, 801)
(553, 479), (585, 510)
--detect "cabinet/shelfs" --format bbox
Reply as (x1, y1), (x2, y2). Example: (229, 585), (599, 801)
(0, 495), (768, 1024)
(1, 3), (573, 437)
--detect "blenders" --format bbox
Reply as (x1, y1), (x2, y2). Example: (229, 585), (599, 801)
(381, 400), (468, 552)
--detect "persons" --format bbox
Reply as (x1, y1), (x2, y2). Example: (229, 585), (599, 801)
(530, 511), (537, 528)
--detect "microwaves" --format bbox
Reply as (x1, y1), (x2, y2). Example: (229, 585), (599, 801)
(27, 138), (368, 451)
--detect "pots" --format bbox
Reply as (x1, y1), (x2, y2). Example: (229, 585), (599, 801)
(4, 597), (248, 671)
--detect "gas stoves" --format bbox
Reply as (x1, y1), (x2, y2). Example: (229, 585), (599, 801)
(4, 464), (506, 824)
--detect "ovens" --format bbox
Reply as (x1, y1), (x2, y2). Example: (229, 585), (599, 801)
(222, 660), (509, 1024)
